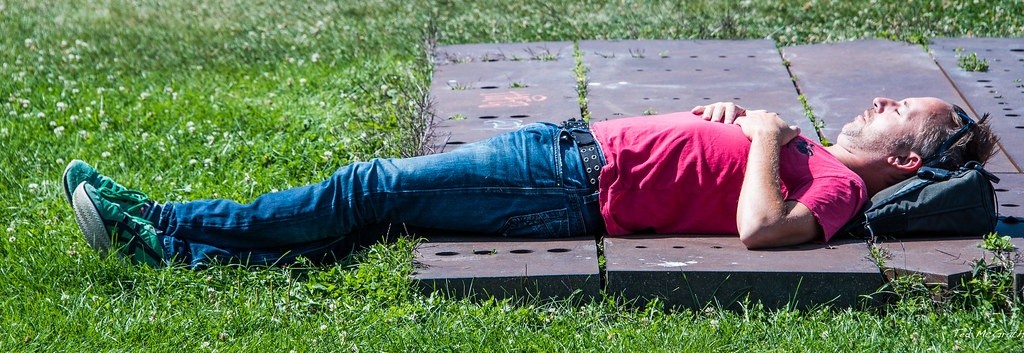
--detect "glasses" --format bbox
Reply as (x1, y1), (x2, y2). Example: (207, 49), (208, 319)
(926, 104), (976, 162)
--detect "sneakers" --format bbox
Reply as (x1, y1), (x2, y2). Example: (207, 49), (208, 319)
(60, 161), (142, 221)
(72, 183), (166, 268)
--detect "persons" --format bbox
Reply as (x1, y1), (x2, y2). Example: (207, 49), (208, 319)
(60, 95), (1003, 269)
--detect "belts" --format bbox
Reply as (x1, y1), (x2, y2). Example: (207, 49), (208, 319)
(566, 120), (603, 191)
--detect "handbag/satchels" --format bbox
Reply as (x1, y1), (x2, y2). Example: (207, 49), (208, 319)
(861, 161), (1000, 237)
